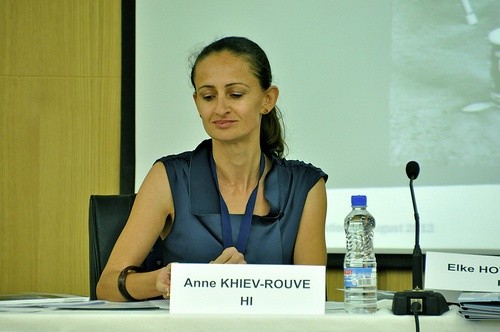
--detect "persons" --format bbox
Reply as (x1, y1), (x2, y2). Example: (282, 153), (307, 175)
(96, 37), (328, 302)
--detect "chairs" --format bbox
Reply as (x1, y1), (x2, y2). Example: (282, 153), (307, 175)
(89, 194), (163, 300)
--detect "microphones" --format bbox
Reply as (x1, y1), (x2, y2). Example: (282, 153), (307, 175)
(391, 161), (449, 316)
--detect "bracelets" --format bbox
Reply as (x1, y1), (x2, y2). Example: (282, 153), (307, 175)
(117, 265), (141, 301)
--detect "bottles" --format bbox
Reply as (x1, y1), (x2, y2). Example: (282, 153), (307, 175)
(344, 195), (377, 314)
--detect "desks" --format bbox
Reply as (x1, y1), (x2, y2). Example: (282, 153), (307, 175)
(0, 296), (500, 332)
